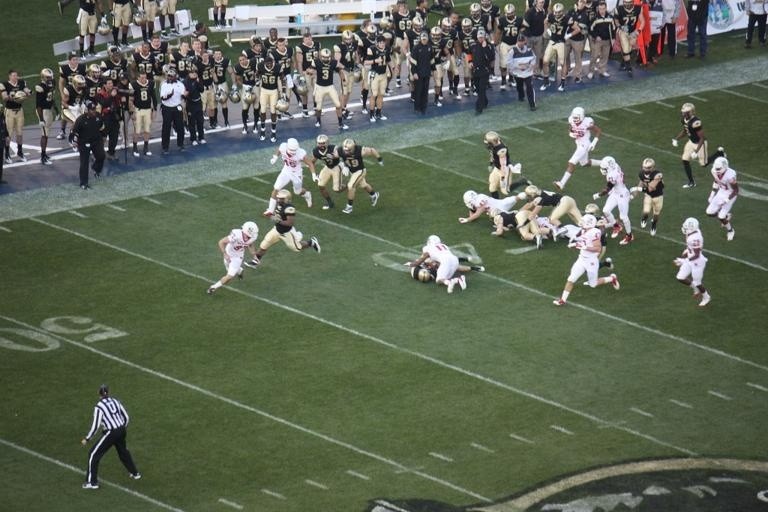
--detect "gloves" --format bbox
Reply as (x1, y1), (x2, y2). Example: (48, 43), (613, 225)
(563, 32), (573, 41)
(592, 193), (601, 200)
(311, 172), (320, 184)
(690, 151), (698, 160)
(587, 137), (599, 153)
(100, 13), (108, 25)
(629, 187), (643, 194)
(403, 262), (412, 267)
(341, 166), (350, 178)
(672, 257), (690, 267)
(270, 155), (278, 165)
(39, 120), (46, 127)
(707, 190), (716, 204)
(672, 138), (678, 148)
(575, 241), (587, 251)
(458, 217), (468, 224)
(469, 61), (474, 70)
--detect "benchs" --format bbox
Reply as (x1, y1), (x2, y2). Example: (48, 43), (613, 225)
(52, 9), (196, 66)
(207, 0), (398, 46)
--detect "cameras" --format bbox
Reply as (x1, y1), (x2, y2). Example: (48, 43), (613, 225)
(113, 88), (138, 96)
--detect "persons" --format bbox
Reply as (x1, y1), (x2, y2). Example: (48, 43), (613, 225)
(242, 189), (321, 270)
(0, 2), (706, 192)
(264, 137), (319, 216)
(80, 383), (142, 490)
(309, 134), (383, 215)
(404, 101), (737, 306)
(745, 0), (768, 44)
(204, 221), (260, 294)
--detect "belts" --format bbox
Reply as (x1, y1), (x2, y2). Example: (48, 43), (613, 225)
(88, 12), (94, 15)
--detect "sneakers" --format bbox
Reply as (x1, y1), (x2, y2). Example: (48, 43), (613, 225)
(553, 181), (563, 192)
(726, 228), (735, 241)
(341, 108), (352, 120)
(80, 52), (86, 61)
(41, 157), (53, 165)
(310, 236), (321, 254)
(458, 275), (466, 290)
(462, 90), (470, 96)
(4, 156), (12, 164)
(252, 125), (259, 134)
(341, 203), (353, 215)
(338, 122), (349, 130)
(433, 100), (442, 107)
(458, 256), (471, 262)
(169, 29), (181, 37)
(302, 111), (310, 118)
(160, 31), (170, 39)
(81, 482), (100, 489)
(374, 112), (388, 120)
(369, 113), (377, 123)
(129, 473), (141, 480)
(192, 140), (198, 146)
(557, 83), (565, 91)
(499, 83), (507, 89)
(17, 154), (27, 162)
(360, 109), (368, 115)
(720, 213), (731, 227)
(610, 273), (620, 290)
(143, 141), (153, 156)
(535, 235), (543, 251)
(344, 104), (354, 115)
(314, 120), (320, 127)
(283, 111), (294, 119)
(610, 222), (623, 239)
(369, 191), (379, 207)
(241, 127), (248, 134)
(198, 139), (207, 144)
(207, 285), (216, 295)
(552, 298), (567, 306)
(260, 123), (266, 131)
(259, 130), (266, 141)
(395, 78), (402, 88)
(304, 191), (312, 208)
(554, 227), (568, 237)
(600, 72), (610, 77)
(56, 131), (65, 139)
(472, 87), (478, 96)
(575, 77), (583, 84)
(508, 79), (517, 87)
(649, 219), (657, 237)
(619, 232), (634, 245)
(539, 82), (551, 91)
(270, 123), (276, 133)
(605, 257), (614, 270)
(270, 132), (277, 143)
(243, 259), (259, 270)
(587, 72), (594, 80)
(640, 215), (648, 229)
(698, 294), (712, 307)
(263, 211), (273, 217)
(471, 266), (485, 272)
(132, 141), (140, 157)
(682, 183), (697, 189)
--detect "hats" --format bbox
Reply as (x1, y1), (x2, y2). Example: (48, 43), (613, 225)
(477, 30), (486, 38)
(166, 69), (176, 74)
(420, 33), (428, 40)
(517, 33), (525, 41)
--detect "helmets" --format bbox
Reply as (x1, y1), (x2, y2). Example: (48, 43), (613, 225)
(286, 137), (299, 157)
(681, 217), (699, 235)
(470, 3), (482, 16)
(316, 134), (329, 149)
(98, 25), (110, 35)
(379, 17), (389, 30)
(228, 89), (241, 103)
(375, 36), (386, 52)
(524, 185), (540, 203)
(483, 131), (501, 148)
(106, 45), (123, 60)
(418, 269), (430, 283)
(40, 68), (54, 87)
(571, 107), (584, 124)
(426, 235), (442, 246)
(275, 100), (289, 112)
(489, 208), (502, 219)
(366, 24), (378, 34)
(12, 90), (27, 104)
(580, 214), (597, 229)
(599, 156), (616, 176)
(552, 3), (564, 16)
(463, 190), (478, 211)
(341, 29), (354, 42)
(437, 17), (452, 31)
(275, 189), (292, 205)
(249, 35), (265, 49)
(642, 158), (655, 175)
(503, 3), (516, 17)
(320, 48), (332, 61)
(84, 97), (97, 110)
(412, 16), (424, 29)
(585, 203), (601, 220)
(341, 139), (356, 155)
(296, 85), (308, 96)
(680, 102), (696, 119)
(461, 17), (473, 36)
(713, 156), (728, 176)
(215, 90), (228, 103)
(242, 92), (256, 104)
(87, 64), (101, 83)
(623, 0), (634, 10)
(241, 221), (259, 238)
(132, 15), (145, 27)
(71, 75), (86, 95)
(430, 27), (442, 44)
